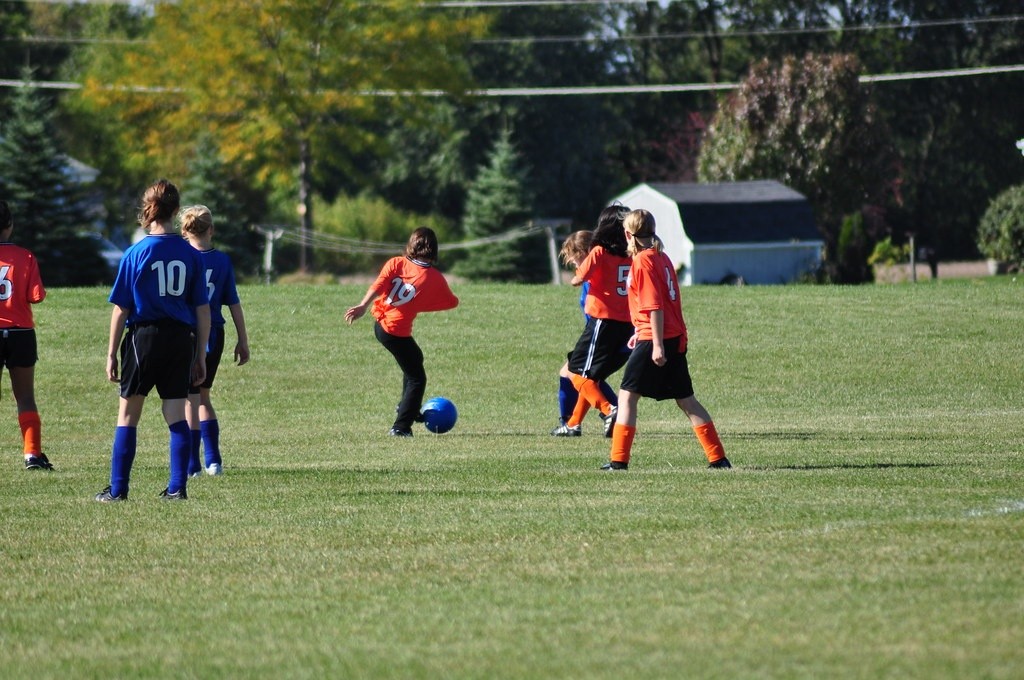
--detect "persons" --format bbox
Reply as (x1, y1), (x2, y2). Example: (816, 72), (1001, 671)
(554, 229), (618, 428)
(180, 205), (249, 478)
(343, 226), (459, 436)
(0, 202), (57, 473)
(549, 205), (635, 437)
(93, 179), (212, 504)
(598, 209), (732, 470)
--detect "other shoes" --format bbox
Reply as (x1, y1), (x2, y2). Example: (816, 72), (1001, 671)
(187, 471), (202, 478)
(599, 460), (629, 470)
(707, 457), (733, 469)
(205, 463), (222, 476)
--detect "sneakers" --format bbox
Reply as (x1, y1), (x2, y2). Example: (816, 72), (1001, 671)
(394, 401), (425, 423)
(549, 423), (581, 437)
(94, 486), (127, 503)
(599, 404), (618, 438)
(389, 428), (413, 436)
(24, 452), (55, 471)
(159, 485), (188, 499)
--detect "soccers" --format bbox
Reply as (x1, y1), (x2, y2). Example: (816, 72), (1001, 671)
(421, 397), (458, 434)
